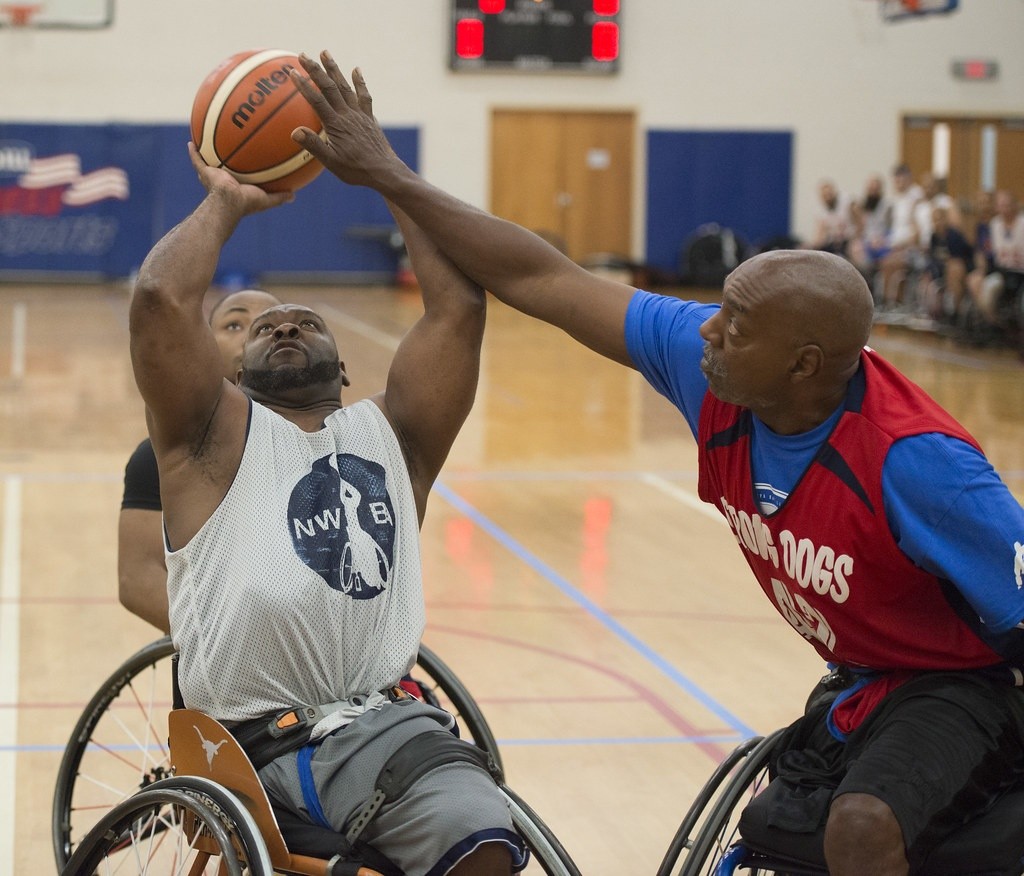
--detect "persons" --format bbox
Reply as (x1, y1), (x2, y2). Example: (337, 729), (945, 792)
(114, 140), (531, 876)
(288, 47), (1024, 876)
(809, 161), (1024, 357)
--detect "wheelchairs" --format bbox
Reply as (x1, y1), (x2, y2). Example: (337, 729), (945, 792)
(652, 676), (1024, 875)
(51, 634), (584, 876)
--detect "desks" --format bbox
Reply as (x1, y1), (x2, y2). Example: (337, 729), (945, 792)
(351, 225), (406, 284)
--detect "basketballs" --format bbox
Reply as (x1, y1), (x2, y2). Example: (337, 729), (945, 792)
(190, 50), (350, 191)
